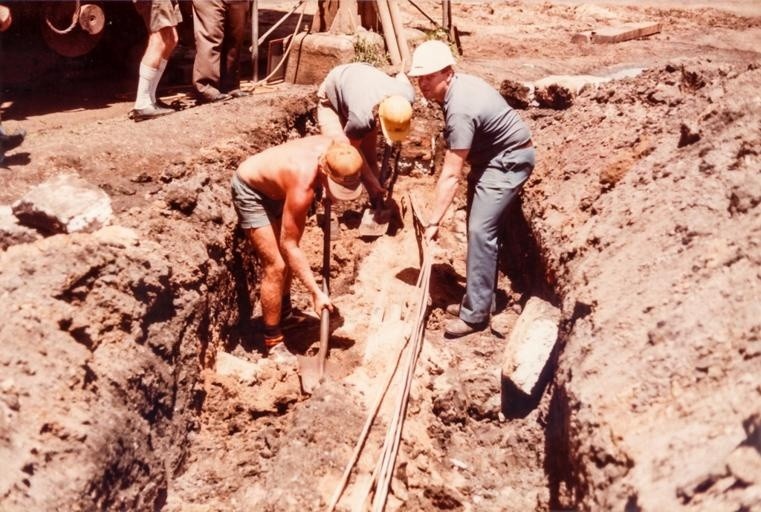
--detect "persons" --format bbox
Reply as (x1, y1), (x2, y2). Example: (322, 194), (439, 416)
(190, 0), (251, 106)
(228, 132), (367, 374)
(406, 39), (538, 338)
(316, 60), (417, 214)
(129, 0), (187, 126)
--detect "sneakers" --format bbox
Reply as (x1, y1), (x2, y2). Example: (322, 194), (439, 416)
(444, 313), (493, 337)
(263, 341), (294, 359)
(282, 311), (320, 332)
(446, 303), (461, 317)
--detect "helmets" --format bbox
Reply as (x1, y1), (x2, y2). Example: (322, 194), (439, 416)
(318, 143), (364, 201)
(379, 96), (413, 142)
(407, 41), (457, 77)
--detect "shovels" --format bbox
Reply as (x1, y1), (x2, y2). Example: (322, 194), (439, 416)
(296, 185), (340, 396)
(358, 142), (394, 238)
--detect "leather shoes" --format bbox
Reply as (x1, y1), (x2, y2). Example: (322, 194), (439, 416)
(131, 98), (180, 122)
(212, 88), (253, 102)
